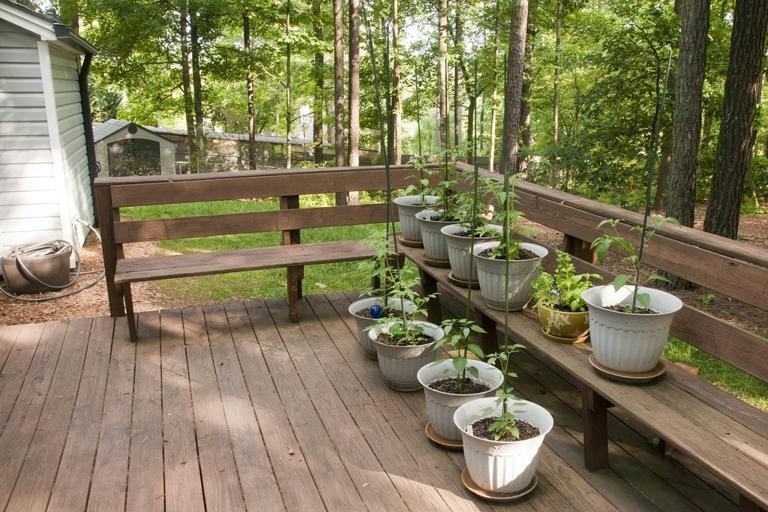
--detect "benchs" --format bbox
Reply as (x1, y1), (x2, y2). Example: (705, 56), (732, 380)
(390, 160), (766, 511)
(108, 165), (447, 347)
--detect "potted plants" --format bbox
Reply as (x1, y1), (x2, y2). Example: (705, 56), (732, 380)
(349, 73), (605, 504)
(579, 213), (683, 384)
